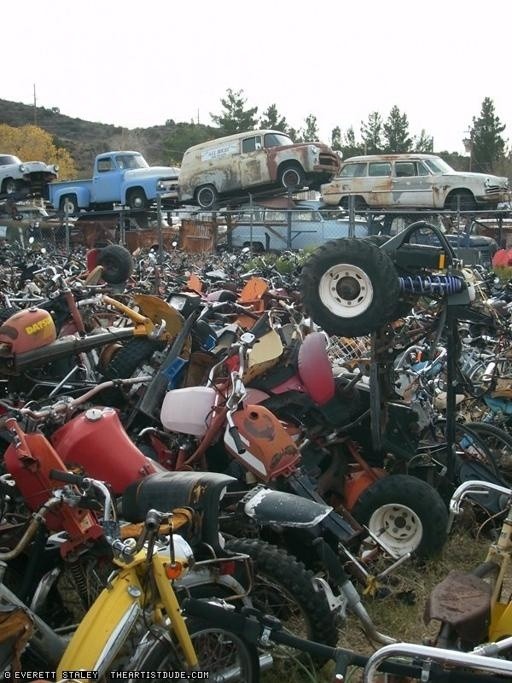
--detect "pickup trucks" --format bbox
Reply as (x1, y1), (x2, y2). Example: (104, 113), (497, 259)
(49, 151), (178, 215)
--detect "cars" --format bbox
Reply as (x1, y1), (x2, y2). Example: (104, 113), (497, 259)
(177, 131), (343, 208)
(322, 155), (509, 211)
(1, 155), (58, 195)
